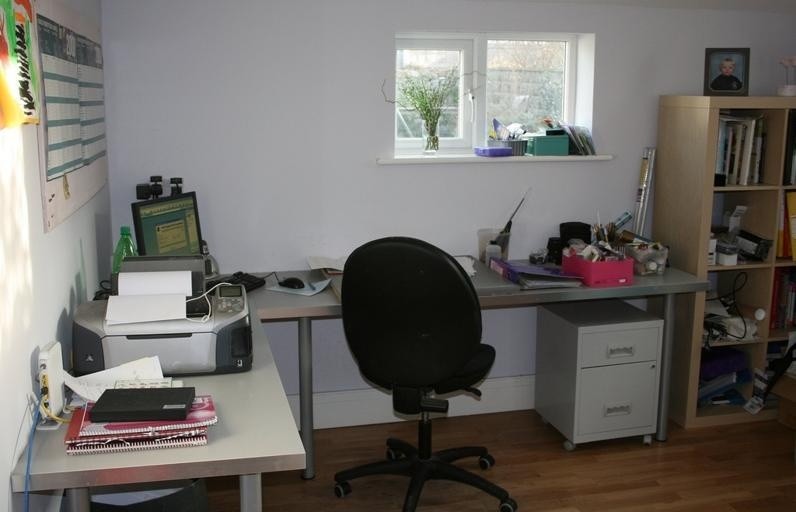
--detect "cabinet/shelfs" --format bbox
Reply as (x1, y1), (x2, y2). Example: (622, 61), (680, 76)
(537, 299), (667, 451)
(644, 96), (796, 432)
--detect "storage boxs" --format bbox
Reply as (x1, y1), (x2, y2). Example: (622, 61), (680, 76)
(557, 250), (636, 288)
(522, 133), (570, 157)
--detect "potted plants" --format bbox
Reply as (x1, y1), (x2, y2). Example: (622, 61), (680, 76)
(394, 66), (484, 153)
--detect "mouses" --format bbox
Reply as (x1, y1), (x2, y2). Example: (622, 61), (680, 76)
(279, 276), (304, 290)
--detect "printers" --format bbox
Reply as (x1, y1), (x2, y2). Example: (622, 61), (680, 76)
(72, 256), (252, 378)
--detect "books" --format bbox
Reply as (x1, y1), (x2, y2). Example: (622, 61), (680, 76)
(771, 267), (796, 332)
(783, 109), (796, 184)
(64, 375), (218, 455)
(777, 189), (796, 261)
(714, 112), (768, 187)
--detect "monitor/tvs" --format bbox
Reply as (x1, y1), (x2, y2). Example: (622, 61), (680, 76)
(130, 191), (203, 257)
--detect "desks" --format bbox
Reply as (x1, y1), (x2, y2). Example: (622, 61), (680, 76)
(8, 251), (713, 512)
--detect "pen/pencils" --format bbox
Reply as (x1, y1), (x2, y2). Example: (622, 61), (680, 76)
(308, 282), (315, 290)
(592, 209), (614, 242)
(482, 197), (526, 261)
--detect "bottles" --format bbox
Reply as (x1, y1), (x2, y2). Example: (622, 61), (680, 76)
(111, 225), (138, 275)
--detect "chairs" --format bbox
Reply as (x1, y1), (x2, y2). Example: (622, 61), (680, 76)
(331, 234), (518, 512)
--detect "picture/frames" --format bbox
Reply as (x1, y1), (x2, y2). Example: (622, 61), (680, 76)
(701, 44), (751, 96)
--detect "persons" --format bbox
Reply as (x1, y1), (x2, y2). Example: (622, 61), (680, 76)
(709, 56), (744, 90)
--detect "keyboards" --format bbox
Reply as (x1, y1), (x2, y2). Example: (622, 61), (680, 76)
(207, 269), (265, 300)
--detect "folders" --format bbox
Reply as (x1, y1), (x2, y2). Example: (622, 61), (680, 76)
(490, 256), (586, 288)
